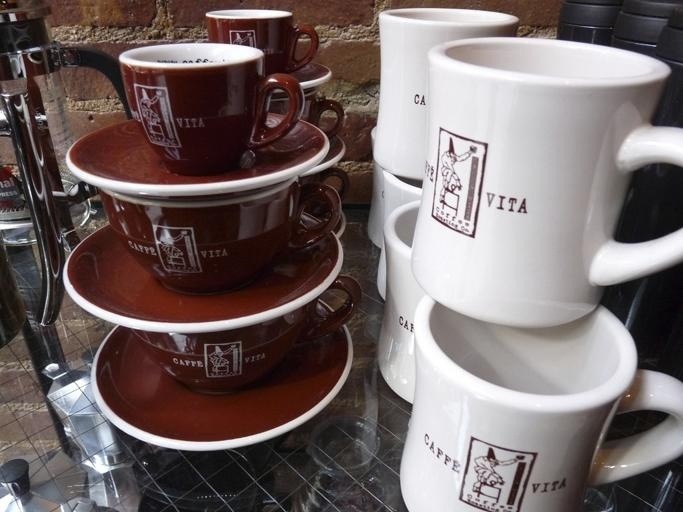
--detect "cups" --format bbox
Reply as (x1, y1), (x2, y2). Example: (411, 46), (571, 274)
(366, 124), (422, 405)
(300, 168), (350, 214)
(300, 85), (346, 140)
(134, 273), (366, 396)
(410, 35), (683, 331)
(397, 297), (683, 511)
(116, 42), (306, 176)
(371, 9), (522, 183)
(97, 179), (344, 297)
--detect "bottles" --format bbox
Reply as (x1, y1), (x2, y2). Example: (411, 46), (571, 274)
(0, 457), (61, 512)
(558, 1), (683, 379)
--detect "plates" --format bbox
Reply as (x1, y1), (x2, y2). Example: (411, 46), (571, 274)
(323, 205), (346, 239)
(89, 299), (355, 454)
(64, 115), (356, 200)
(267, 61), (334, 90)
(60, 211), (345, 336)
